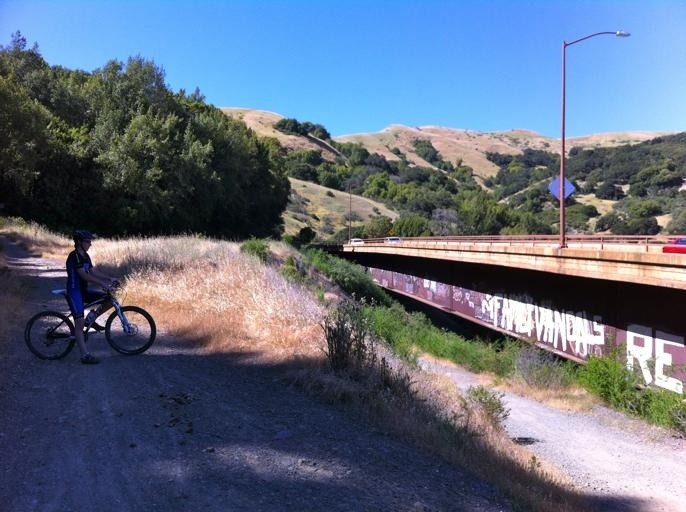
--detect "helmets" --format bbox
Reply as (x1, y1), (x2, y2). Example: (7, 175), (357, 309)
(70, 228), (96, 242)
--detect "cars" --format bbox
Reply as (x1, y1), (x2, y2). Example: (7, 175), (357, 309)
(384, 237), (401, 243)
(348, 238), (364, 245)
(662, 238), (686, 254)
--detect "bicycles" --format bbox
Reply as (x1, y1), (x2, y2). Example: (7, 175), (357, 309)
(23, 280), (156, 360)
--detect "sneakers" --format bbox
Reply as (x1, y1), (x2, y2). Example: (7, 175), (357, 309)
(80, 351), (102, 365)
(83, 319), (105, 331)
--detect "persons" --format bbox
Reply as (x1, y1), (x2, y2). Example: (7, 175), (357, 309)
(65, 227), (123, 365)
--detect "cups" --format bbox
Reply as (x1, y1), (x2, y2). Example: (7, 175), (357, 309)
(85, 309), (95, 326)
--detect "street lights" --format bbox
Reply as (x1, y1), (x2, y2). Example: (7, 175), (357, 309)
(560, 30), (630, 248)
(344, 163), (352, 240)
